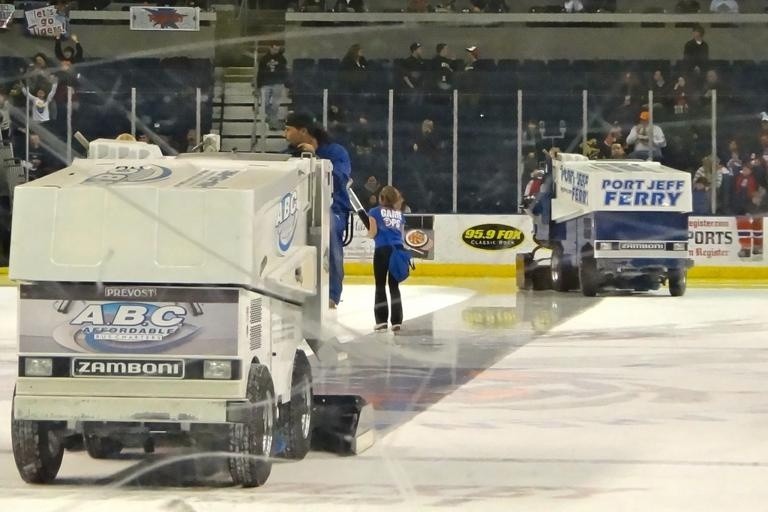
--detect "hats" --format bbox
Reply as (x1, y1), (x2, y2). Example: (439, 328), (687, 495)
(410, 42), (423, 52)
(437, 42), (447, 52)
(640, 111), (649, 120)
(466, 46), (480, 57)
(285, 111), (316, 125)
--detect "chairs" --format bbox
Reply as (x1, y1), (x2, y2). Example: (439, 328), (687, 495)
(2, 57), (768, 214)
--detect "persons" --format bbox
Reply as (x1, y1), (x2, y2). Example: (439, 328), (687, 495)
(280, 113), (351, 308)
(257, 40), (286, 129)
(0, 0), (211, 172)
(368, 185), (406, 331)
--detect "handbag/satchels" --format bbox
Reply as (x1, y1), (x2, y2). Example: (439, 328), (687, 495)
(388, 248), (411, 282)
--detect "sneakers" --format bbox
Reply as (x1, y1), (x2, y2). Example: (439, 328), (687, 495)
(391, 323), (401, 331)
(374, 323), (388, 331)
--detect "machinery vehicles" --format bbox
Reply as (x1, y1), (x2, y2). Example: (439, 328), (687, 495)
(514, 150), (695, 297)
(7, 136), (379, 490)
